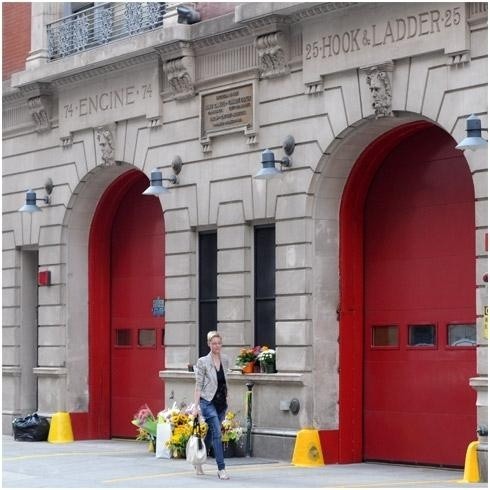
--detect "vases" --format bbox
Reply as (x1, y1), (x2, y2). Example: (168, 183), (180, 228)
(262, 359), (274, 374)
(242, 361), (254, 373)
(210, 438), (236, 458)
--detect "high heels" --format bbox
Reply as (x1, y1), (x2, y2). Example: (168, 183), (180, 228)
(217, 470), (230, 480)
(193, 465), (204, 475)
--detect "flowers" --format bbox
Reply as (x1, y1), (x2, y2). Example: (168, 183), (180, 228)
(257, 349), (276, 362)
(235, 346), (269, 368)
(220, 412), (242, 442)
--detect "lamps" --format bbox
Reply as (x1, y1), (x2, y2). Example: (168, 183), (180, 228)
(16, 177), (54, 213)
(454, 114), (487, 151)
(253, 134), (295, 181)
(142, 155), (182, 197)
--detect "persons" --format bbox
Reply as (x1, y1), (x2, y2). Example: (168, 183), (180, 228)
(189, 331), (235, 483)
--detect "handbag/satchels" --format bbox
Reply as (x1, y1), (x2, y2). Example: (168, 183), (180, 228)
(186, 435), (207, 465)
